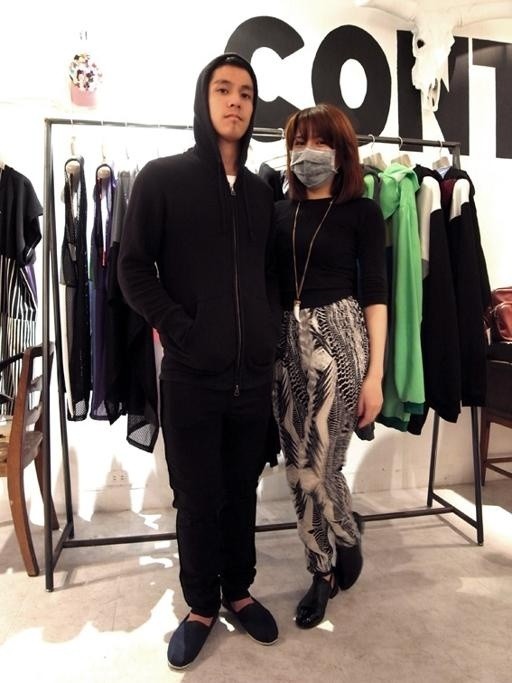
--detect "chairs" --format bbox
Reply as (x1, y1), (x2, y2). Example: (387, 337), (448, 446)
(0, 343), (59, 579)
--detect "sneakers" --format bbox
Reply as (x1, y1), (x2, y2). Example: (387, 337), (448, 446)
(222, 597), (279, 645)
(168, 614), (218, 670)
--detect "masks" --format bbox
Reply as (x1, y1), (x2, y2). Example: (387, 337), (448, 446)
(289, 147), (338, 188)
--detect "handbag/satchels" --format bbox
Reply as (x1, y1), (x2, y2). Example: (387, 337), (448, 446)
(491, 288), (512, 344)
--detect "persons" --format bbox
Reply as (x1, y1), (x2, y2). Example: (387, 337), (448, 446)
(118, 52), (280, 669)
(274, 103), (387, 629)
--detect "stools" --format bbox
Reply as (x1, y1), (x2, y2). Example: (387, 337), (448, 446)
(481, 342), (512, 487)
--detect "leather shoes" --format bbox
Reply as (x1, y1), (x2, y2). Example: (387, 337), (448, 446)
(297, 575), (339, 629)
(337, 512), (364, 589)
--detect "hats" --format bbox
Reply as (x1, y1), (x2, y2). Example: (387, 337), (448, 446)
(69, 53), (101, 107)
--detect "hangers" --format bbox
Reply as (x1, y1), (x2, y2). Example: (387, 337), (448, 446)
(41, 118), (484, 593)
